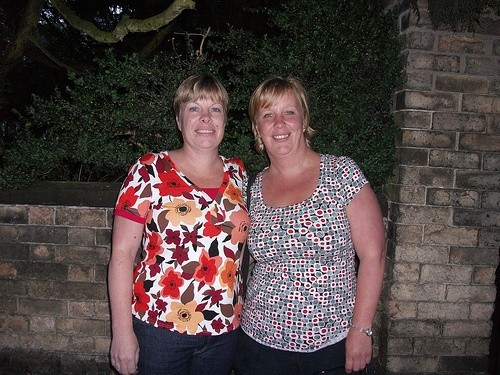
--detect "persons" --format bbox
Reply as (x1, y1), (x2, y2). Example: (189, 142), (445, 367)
(106, 74), (253, 375)
(235, 75), (385, 375)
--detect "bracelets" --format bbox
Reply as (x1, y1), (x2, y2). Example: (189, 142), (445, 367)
(349, 321), (375, 342)
